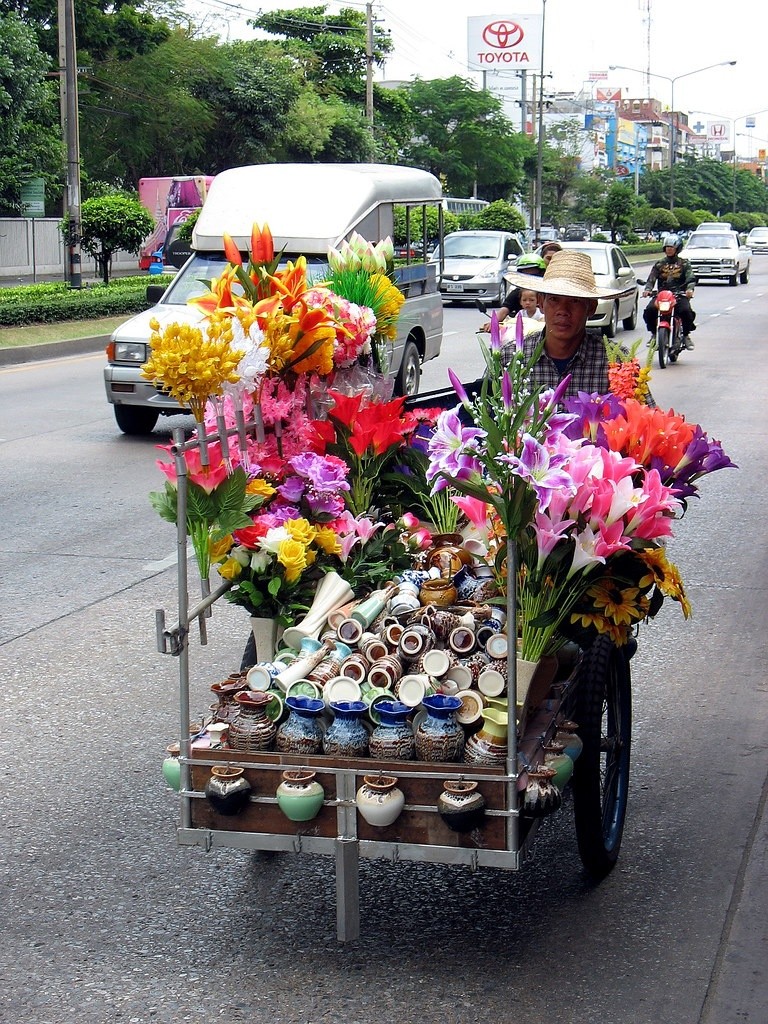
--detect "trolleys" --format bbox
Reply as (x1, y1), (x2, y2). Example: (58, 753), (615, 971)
(154, 406), (637, 941)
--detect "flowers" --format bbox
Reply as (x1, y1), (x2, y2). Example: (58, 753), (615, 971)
(140, 221), (738, 661)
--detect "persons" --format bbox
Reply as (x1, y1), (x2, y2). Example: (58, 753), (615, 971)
(485, 251), (655, 418)
(482, 243), (561, 333)
(643, 234), (697, 351)
(645, 233), (654, 242)
(499, 288), (544, 331)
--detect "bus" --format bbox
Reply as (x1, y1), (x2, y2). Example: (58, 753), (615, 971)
(443, 197), (491, 216)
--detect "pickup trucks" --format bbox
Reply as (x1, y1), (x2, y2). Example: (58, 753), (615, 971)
(103, 164), (444, 433)
(677, 230), (752, 286)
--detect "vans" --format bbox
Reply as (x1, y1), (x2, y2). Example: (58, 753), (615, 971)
(688, 223), (734, 238)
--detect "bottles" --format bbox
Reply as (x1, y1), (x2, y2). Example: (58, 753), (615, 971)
(162, 540), (583, 835)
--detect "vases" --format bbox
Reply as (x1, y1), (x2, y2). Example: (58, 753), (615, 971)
(276, 770), (324, 821)
(542, 741), (574, 793)
(521, 766), (562, 819)
(205, 766), (252, 817)
(437, 781), (485, 833)
(190, 532), (558, 765)
(161, 744), (181, 792)
(555, 720), (583, 761)
(356, 774), (404, 827)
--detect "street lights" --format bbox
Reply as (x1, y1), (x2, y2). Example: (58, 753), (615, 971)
(686, 109), (767, 212)
(609, 61), (737, 213)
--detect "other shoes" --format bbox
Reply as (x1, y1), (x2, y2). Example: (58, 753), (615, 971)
(683, 335), (694, 350)
(647, 337), (655, 347)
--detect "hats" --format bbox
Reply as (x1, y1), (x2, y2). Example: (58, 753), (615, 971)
(503, 250), (636, 301)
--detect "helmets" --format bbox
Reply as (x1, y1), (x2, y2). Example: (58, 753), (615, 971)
(663, 234), (683, 255)
(516, 253), (547, 275)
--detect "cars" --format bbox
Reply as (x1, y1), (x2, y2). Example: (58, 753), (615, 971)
(518, 221), (693, 253)
(746, 227), (768, 253)
(507, 242), (640, 337)
(149, 220), (195, 277)
(429, 231), (526, 308)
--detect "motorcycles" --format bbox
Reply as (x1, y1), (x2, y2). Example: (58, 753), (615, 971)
(640, 277), (699, 369)
